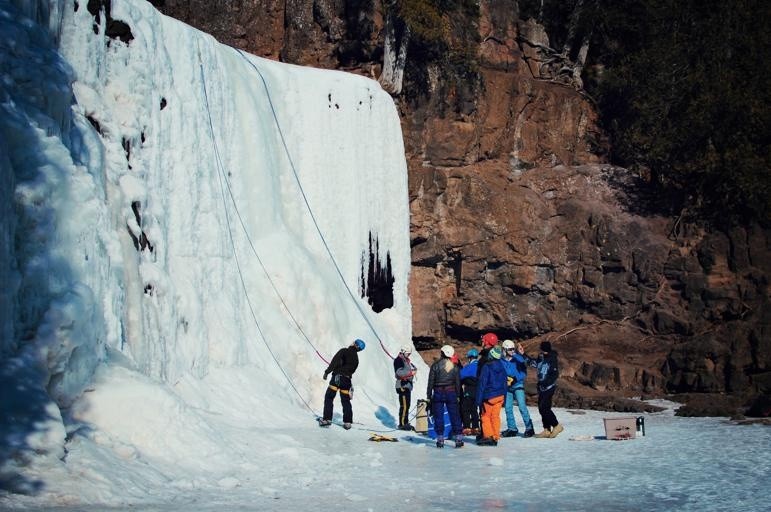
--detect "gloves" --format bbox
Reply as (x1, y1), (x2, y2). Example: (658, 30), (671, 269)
(506, 377), (516, 386)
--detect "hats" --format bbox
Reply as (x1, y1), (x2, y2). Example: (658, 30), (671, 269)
(539, 342), (550, 352)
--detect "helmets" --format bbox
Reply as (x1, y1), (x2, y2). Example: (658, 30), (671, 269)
(354, 333), (515, 360)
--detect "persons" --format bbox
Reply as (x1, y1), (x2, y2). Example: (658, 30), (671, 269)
(501, 339), (536, 437)
(478, 332), (498, 375)
(452, 353), (464, 368)
(459, 347), (480, 436)
(394, 343), (418, 428)
(474, 345), (509, 447)
(517, 341), (564, 438)
(319, 338), (366, 429)
(427, 344), (466, 448)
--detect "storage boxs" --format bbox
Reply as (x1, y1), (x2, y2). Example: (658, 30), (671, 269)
(602, 417), (638, 441)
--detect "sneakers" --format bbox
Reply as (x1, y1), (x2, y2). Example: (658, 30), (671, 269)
(319, 420), (331, 425)
(344, 423), (350, 429)
(398, 423), (563, 448)
